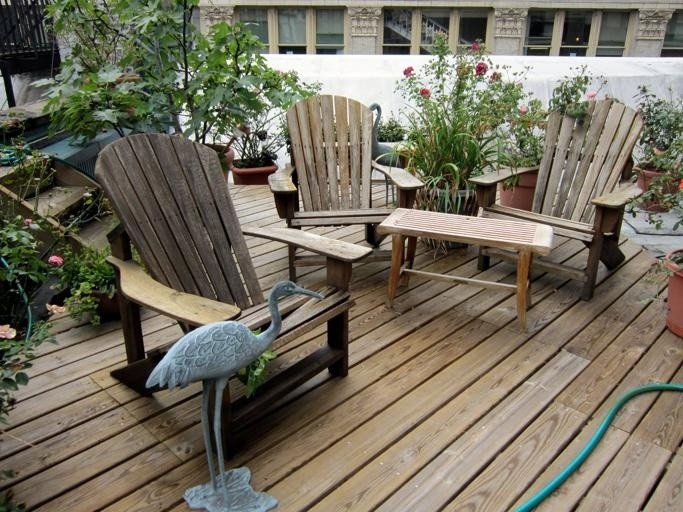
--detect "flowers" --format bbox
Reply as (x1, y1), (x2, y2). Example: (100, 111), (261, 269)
(0, 219), (120, 442)
(403, 34), (683, 172)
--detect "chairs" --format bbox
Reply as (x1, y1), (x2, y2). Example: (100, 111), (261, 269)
(93, 134), (371, 442)
(269, 95), (429, 287)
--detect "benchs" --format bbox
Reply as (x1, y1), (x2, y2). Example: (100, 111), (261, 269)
(472, 97), (650, 304)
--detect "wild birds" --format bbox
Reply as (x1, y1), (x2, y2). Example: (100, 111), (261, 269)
(368, 103), (404, 168)
(145, 281), (324, 504)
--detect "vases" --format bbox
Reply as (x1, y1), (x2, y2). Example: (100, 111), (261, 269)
(665, 244), (683, 337)
(635, 165), (680, 212)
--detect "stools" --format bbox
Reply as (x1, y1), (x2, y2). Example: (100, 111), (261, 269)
(377, 206), (558, 335)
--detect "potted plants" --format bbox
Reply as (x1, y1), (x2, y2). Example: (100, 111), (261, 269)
(187, 54), (316, 184)
(177, 23), (271, 178)
